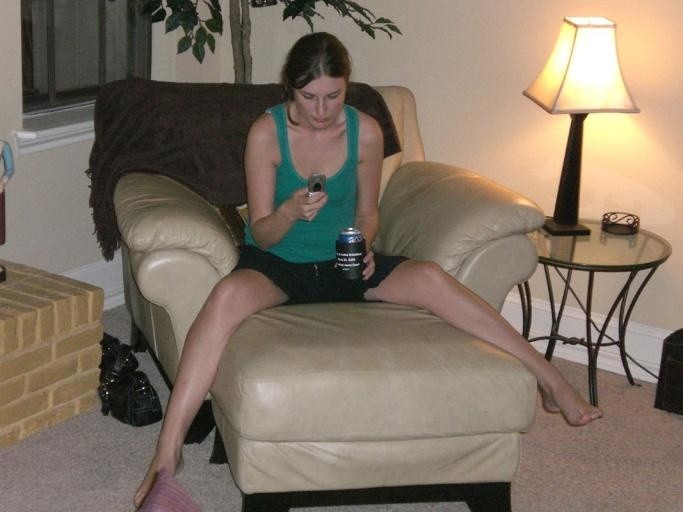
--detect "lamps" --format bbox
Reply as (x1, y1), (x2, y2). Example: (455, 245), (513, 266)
(520, 16), (641, 236)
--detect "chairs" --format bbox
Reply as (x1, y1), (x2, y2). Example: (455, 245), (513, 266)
(93, 77), (546, 511)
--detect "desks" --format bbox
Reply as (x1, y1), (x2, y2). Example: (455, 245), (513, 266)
(516, 217), (672, 406)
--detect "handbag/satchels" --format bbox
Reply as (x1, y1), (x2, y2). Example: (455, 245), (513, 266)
(98, 332), (163, 427)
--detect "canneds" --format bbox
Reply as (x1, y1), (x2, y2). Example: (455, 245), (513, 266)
(339, 228), (363, 243)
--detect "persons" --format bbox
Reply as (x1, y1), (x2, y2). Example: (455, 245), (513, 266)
(133, 31), (603, 510)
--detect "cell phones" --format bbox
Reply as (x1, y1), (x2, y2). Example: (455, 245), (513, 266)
(308, 174), (325, 192)
